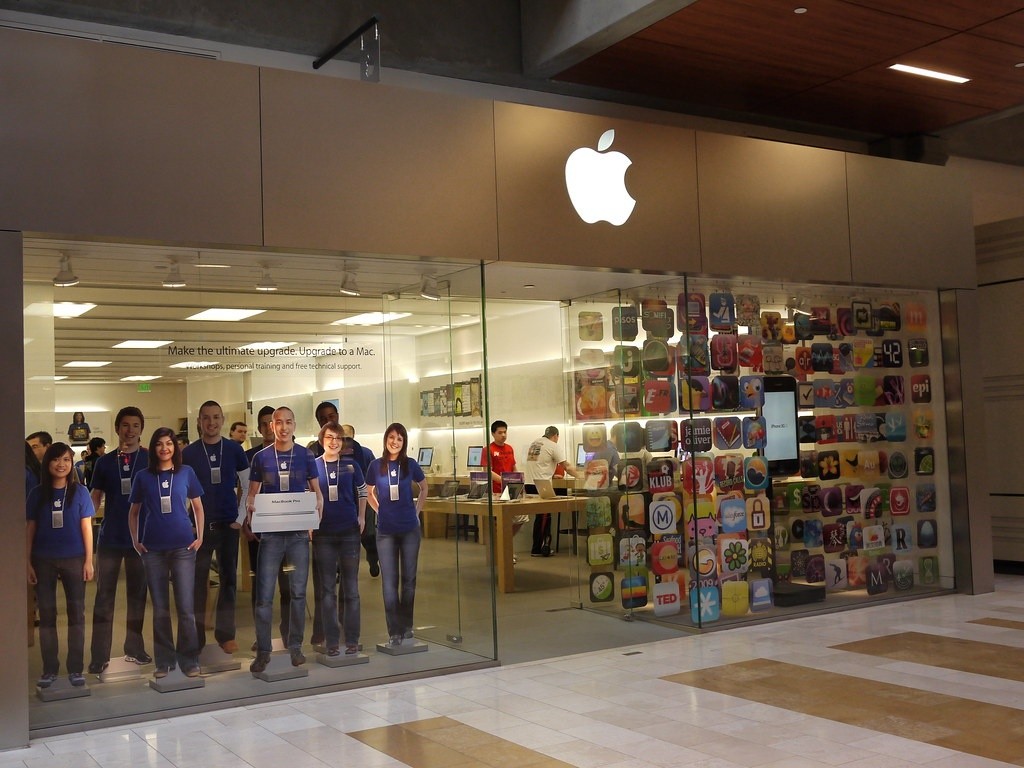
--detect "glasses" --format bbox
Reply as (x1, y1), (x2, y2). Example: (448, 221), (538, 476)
(324, 435), (343, 441)
(319, 413), (337, 422)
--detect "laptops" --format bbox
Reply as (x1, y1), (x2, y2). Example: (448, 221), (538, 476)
(425, 480), (573, 503)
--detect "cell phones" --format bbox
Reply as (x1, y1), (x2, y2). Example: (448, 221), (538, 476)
(420, 375), (483, 418)
(762, 374), (801, 480)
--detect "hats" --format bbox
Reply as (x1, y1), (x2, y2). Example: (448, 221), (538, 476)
(542, 426), (559, 436)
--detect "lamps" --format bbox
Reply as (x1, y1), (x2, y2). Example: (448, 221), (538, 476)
(162, 256), (186, 288)
(419, 273), (441, 301)
(53, 256), (80, 287)
(256, 261), (278, 291)
(339, 270), (360, 296)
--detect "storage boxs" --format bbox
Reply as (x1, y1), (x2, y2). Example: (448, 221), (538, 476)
(251, 492), (319, 533)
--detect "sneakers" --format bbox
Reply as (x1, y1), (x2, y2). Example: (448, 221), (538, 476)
(327, 646), (340, 655)
(88, 659), (109, 675)
(389, 634), (402, 644)
(346, 645), (357, 652)
(250, 653), (271, 672)
(69, 672), (85, 686)
(124, 651), (152, 664)
(37, 673), (58, 690)
(404, 627), (414, 638)
(292, 648), (306, 665)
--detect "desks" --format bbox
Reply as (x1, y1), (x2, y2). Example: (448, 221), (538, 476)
(411, 473), (653, 569)
(412, 494), (589, 594)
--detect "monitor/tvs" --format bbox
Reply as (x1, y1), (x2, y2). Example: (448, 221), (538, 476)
(467, 446), (484, 467)
(418, 447), (434, 467)
(576, 444), (586, 468)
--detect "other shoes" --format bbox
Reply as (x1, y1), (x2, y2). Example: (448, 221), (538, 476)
(540, 534), (551, 556)
(219, 639), (238, 652)
(153, 662), (175, 677)
(210, 580), (219, 587)
(531, 549), (554, 556)
(177, 659), (200, 676)
(369, 561), (380, 577)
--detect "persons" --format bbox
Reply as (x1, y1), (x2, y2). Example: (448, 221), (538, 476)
(590, 422), (625, 487)
(67, 412), (91, 447)
(621, 427), (654, 489)
(365, 424), (428, 644)
(246, 406), (323, 673)
(75, 437), (106, 492)
(26, 442), (94, 687)
(341, 424), (380, 577)
(174, 433), (189, 464)
(88, 407), (150, 674)
(523, 426), (584, 556)
(25, 431), (53, 498)
(137, 437), (149, 452)
(128, 427), (205, 678)
(308, 401), (366, 490)
(237, 405), (291, 651)
(179, 400), (250, 655)
(307, 422), (369, 657)
(228, 422), (248, 445)
(480, 421), (529, 564)
